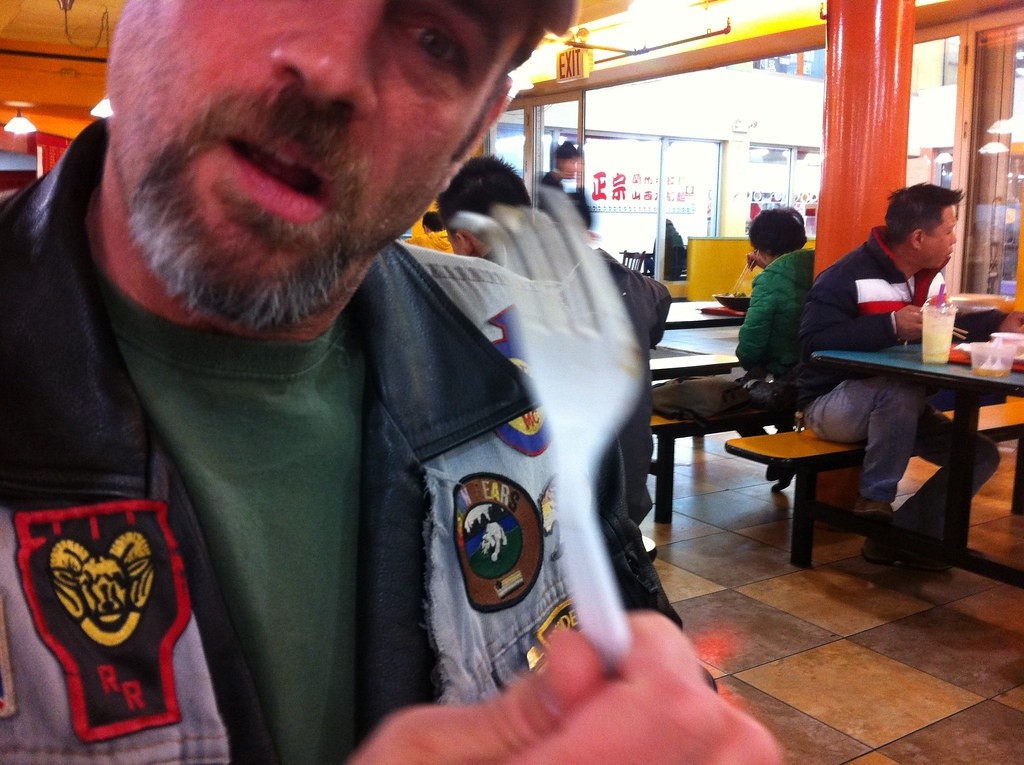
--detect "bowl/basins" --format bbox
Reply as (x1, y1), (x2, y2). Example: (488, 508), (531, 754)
(990, 332), (1024, 362)
(970, 341), (1017, 378)
(711, 294), (751, 312)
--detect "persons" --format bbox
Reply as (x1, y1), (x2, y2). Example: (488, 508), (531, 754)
(542, 142), (600, 244)
(437, 157), (671, 524)
(735, 210), (815, 481)
(792, 184), (1001, 568)
(0, 1), (780, 765)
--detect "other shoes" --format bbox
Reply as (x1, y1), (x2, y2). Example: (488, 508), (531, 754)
(862, 550), (952, 571)
(853, 496), (895, 522)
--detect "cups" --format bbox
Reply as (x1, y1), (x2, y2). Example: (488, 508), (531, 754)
(921, 293), (959, 366)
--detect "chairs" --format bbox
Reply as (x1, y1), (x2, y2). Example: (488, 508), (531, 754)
(622, 250), (645, 272)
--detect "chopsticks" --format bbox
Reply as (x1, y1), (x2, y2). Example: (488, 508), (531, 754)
(952, 327), (967, 340)
(731, 250), (759, 296)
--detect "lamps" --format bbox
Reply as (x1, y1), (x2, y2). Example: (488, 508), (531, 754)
(4, 110), (38, 134)
(90, 95), (114, 118)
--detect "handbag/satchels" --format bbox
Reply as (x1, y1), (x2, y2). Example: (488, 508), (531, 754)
(649, 376), (749, 427)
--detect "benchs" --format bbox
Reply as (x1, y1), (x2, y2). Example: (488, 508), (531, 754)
(649, 354), (801, 524)
(726, 395), (1024, 568)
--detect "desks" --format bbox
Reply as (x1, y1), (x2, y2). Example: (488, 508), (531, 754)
(810, 340), (1024, 587)
(665, 301), (745, 330)
(619, 252), (654, 276)
